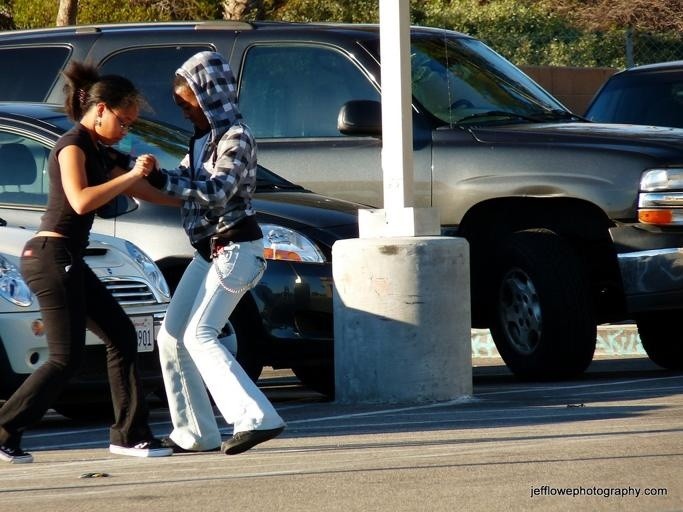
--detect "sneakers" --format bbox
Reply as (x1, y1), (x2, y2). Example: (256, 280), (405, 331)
(222, 426), (285, 456)
(110, 437), (220, 457)
(0, 445), (35, 464)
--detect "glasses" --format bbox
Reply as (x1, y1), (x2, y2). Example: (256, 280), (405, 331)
(110, 110), (135, 132)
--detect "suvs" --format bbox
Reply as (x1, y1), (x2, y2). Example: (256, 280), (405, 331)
(0, 20), (683, 392)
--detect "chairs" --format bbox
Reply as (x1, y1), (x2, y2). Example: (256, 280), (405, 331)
(0, 143), (47, 205)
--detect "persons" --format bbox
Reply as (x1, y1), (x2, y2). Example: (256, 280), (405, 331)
(0, 59), (173, 464)
(142, 51), (287, 456)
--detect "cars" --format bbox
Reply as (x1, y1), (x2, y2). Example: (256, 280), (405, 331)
(574, 57), (683, 131)
(0, 99), (377, 396)
(0, 221), (174, 400)
